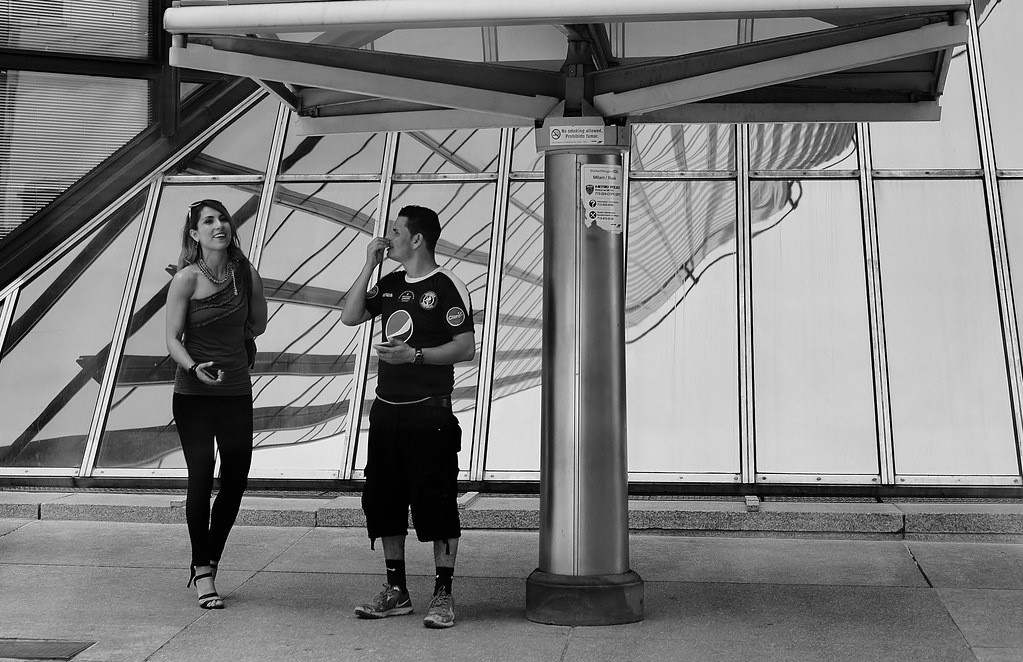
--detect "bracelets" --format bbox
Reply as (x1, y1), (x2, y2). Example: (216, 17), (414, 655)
(189, 363), (198, 378)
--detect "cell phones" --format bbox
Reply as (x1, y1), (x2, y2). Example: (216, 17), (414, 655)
(201, 368), (218, 380)
(377, 342), (394, 348)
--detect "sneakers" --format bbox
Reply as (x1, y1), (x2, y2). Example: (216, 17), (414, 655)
(423, 585), (455, 628)
(354, 586), (414, 617)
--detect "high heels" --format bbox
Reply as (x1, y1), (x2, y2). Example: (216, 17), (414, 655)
(187, 563), (224, 609)
(187, 562), (217, 588)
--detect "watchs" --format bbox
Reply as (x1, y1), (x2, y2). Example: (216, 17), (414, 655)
(413, 347), (424, 365)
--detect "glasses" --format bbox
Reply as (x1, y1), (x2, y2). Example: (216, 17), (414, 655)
(188, 199), (223, 224)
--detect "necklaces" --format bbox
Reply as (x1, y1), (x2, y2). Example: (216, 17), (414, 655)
(196, 256), (231, 284)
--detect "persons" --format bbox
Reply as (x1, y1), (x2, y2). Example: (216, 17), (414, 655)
(341, 206), (476, 629)
(165, 199), (267, 609)
